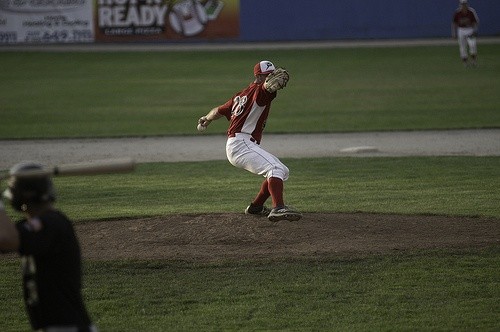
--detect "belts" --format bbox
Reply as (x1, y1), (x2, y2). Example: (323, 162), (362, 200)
(229, 132), (255, 142)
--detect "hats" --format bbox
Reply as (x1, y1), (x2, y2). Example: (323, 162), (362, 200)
(253, 61), (276, 76)
(3, 162), (53, 199)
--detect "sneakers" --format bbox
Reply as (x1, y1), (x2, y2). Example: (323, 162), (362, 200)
(267, 205), (302, 222)
(245, 203), (272, 217)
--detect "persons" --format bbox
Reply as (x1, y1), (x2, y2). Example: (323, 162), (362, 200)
(197, 61), (304, 223)
(451, 0), (480, 69)
(0, 162), (91, 332)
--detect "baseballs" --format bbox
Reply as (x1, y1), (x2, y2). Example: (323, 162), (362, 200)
(197, 123), (207, 131)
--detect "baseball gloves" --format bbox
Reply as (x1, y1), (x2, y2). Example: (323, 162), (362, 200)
(266, 67), (289, 92)
